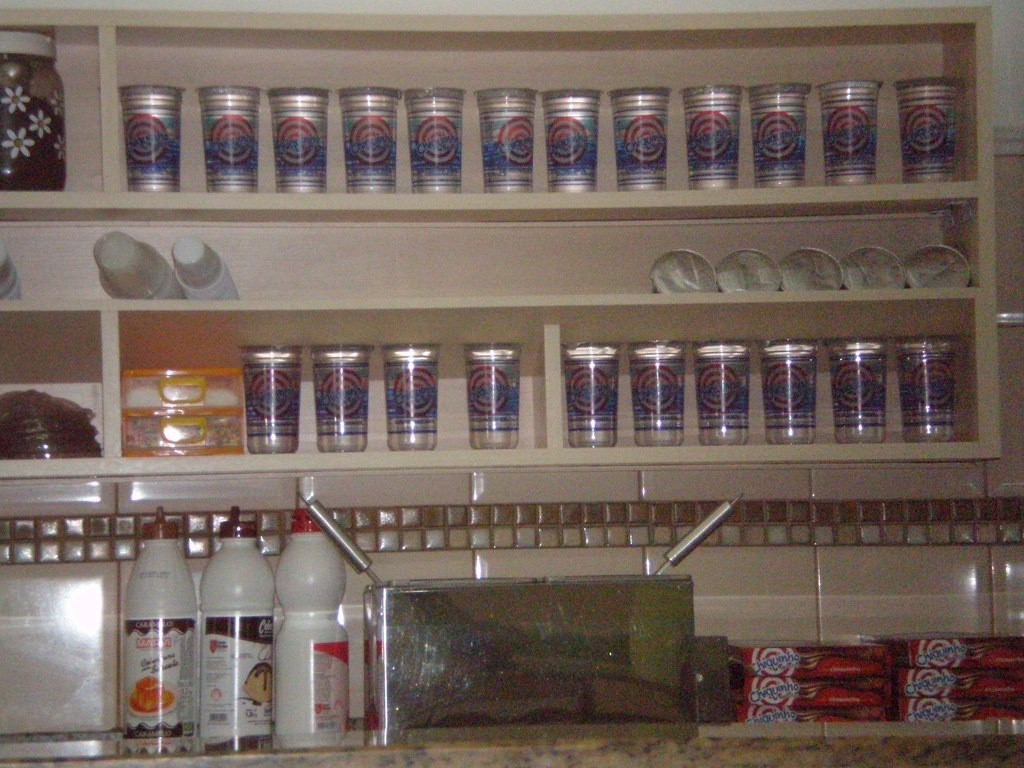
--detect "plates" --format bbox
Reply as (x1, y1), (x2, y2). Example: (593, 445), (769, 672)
(649, 244), (970, 294)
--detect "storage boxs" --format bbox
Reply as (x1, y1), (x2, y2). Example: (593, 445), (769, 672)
(363, 576), (696, 746)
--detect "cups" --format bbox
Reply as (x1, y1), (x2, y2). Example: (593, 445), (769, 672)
(816, 81), (879, 187)
(745, 82), (813, 189)
(196, 86), (263, 192)
(95, 230), (242, 299)
(404, 87), (467, 191)
(606, 85), (671, 191)
(897, 76), (963, 184)
(264, 87), (331, 194)
(475, 87), (537, 193)
(334, 86), (401, 193)
(242, 331), (963, 453)
(679, 85), (744, 192)
(118, 85), (185, 193)
(538, 89), (601, 191)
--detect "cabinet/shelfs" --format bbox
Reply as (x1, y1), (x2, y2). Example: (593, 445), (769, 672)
(0, 0), (1024, 766)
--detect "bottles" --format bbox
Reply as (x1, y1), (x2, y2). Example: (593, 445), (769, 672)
(0, 29), (67, 193)
(119, 506), (352, 759)
